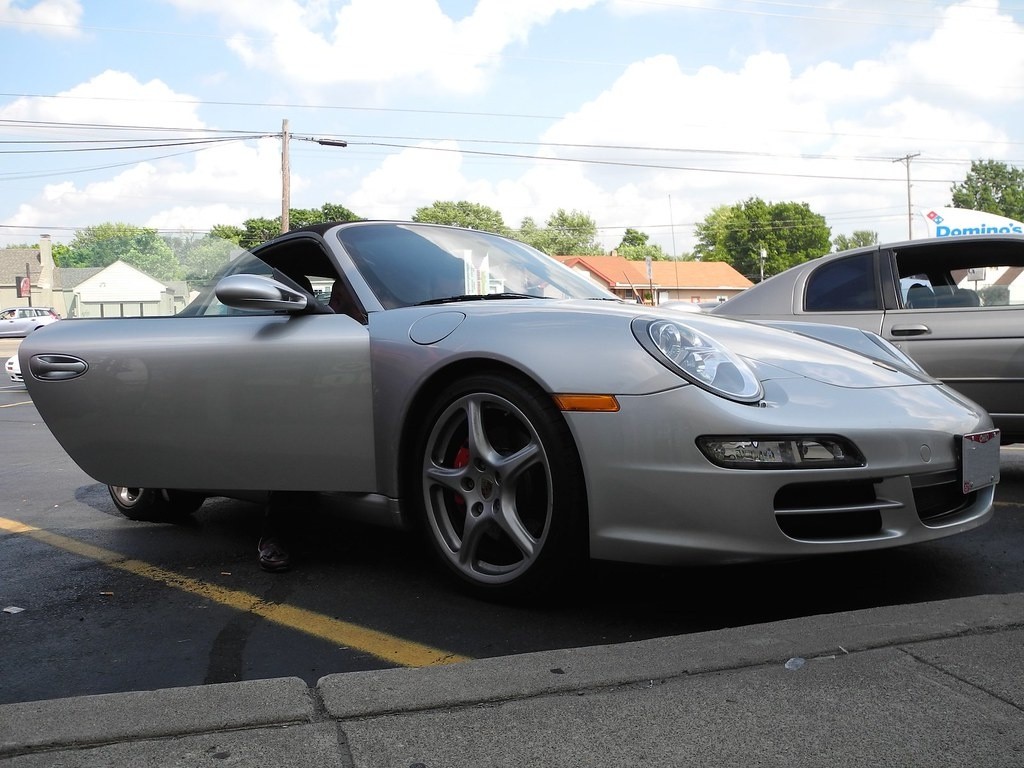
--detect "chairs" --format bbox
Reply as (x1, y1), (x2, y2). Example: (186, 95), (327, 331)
(907, 283), (937, 308)
(329, 276), (401, 322)
(266, 270), (314, 317)
(949, 289), (980, 307)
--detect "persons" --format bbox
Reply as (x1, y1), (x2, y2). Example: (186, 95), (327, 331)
(9, 311), (15, 318)
(255, 271), (404, 572)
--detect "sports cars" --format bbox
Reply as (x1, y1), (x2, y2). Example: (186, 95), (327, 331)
(18, 219), (1001, 604)
(5, 354), (24, 382)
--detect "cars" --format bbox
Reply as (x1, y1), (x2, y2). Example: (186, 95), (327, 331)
(0, 307), (61, 337)
(709, 233), (1024, 444)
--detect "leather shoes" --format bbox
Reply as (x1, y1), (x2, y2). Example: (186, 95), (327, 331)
(256, 536), (291, 571)
